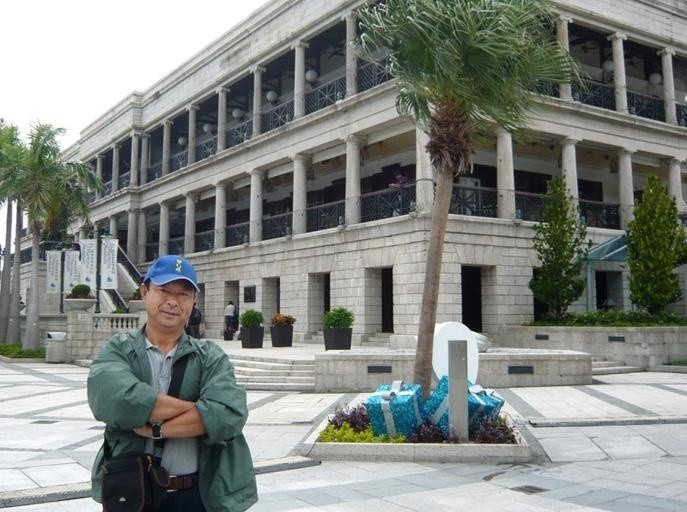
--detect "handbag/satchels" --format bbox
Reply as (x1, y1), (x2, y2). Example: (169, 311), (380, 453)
(100, 451), (174, 512)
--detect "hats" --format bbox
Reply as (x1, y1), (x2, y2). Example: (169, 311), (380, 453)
(143, 253), (201, 295)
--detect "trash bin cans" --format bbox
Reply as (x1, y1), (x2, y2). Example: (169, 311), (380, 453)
(45, 332), (67, 362)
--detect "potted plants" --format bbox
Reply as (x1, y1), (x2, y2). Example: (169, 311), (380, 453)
(238, 310), (264, 350)
(270, 313), (297, 348)
(323, 308), (354, 351)
(64, 284), (98, 310)
(129, 288), (146, 311)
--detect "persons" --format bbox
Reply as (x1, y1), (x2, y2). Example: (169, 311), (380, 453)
(222, 300), (236, 341)
(86, 253), (259, 512)
(186, 301), (203, 339)
(388, 171), (410, 216)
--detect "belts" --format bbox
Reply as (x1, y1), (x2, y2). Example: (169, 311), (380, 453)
(168, 473), (199, 493)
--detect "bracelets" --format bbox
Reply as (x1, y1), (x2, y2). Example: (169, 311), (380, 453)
(149, 421), (164, 443)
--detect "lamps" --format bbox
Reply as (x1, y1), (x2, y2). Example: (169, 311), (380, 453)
(233, 107), (244, 120)
(203, 123), (216, 134)
(178, 136), (187, 146)
(306, 69), (318, 82)
(649, 72), (662, 85)
(267, 90), (278, 103)
(603, 59), (615, 73)
